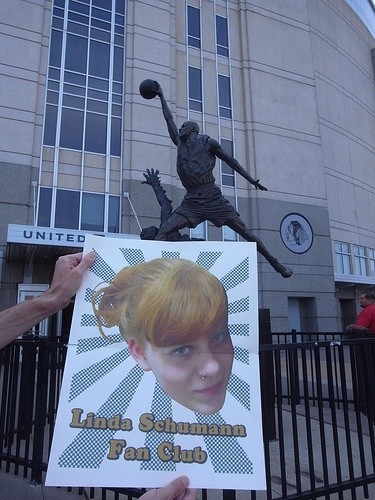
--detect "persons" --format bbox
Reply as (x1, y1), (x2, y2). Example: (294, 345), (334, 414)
(140, 80), (294, 278)
(92, 257), (234, 415)
(0, 251), (198, 500)
(346, 288), (375, 336)
